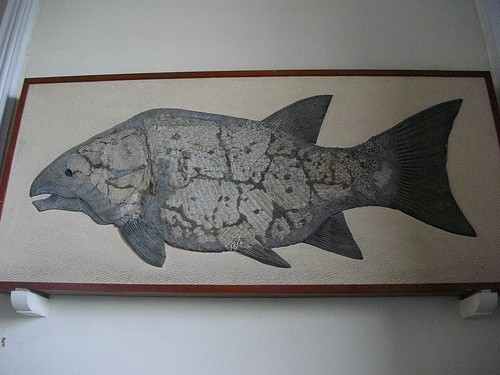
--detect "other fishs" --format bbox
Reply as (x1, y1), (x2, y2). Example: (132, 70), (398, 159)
(29, 92), (479, 271)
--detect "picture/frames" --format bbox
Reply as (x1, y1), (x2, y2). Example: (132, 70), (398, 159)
(0, 69), (500, 298)
